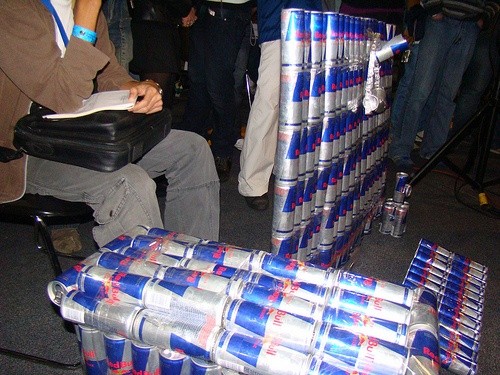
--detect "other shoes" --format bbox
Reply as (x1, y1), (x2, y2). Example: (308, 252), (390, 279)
(246, 194), (268, 210)
(214, 156), (231, 183)
(391, 154), (430, 169)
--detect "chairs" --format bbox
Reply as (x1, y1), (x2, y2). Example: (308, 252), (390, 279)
(131, 20), (188, 124)
(0, 175), (168, 370)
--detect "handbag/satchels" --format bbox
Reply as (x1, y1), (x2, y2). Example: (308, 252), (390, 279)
(13, 103), (171, 173)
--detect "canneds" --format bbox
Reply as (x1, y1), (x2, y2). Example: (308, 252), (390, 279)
(47, 9), (489, 375)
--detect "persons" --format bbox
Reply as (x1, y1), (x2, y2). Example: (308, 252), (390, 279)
(373, 0), (499, 179)
(103, 0), (257, 181)
(236, 0), (325, 213)
(0, 0), (220, 247)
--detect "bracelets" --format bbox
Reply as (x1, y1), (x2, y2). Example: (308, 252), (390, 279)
(72, 26), (98, 45)
